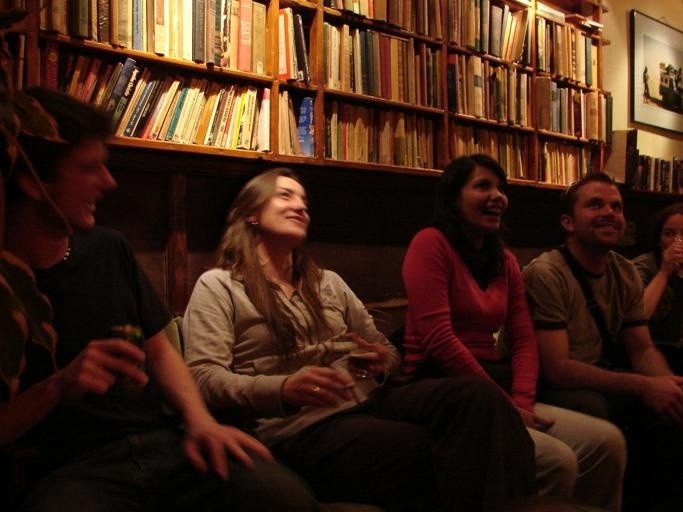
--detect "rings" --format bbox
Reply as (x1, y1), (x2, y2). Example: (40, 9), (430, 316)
(310, 386), (319, 396)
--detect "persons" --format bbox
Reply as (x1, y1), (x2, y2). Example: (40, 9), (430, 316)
(0, 86), (322, 512)
(396, 153), (627, 510)
(521, 169), (681, 510)
(182, 167), (539, 511)
(642, 66), (650, 104)
(674, 66), (683, 114)
(627, 200), (682, 378)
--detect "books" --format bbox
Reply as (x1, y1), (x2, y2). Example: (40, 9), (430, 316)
(630, 148), (682, 194)
(1, 0), (315, 156)
(323, 1), (614, 184)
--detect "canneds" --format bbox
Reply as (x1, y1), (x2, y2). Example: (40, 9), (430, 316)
(110, 328), (145, 386)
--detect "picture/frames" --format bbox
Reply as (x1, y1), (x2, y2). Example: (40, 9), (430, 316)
(631, 8), (683, 133)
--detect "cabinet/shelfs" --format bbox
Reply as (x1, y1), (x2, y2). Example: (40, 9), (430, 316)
(0, 0), (611, 189)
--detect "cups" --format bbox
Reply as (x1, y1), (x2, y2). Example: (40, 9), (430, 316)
(673, 237), (682, 270)
(345, 308), (393, 382)
